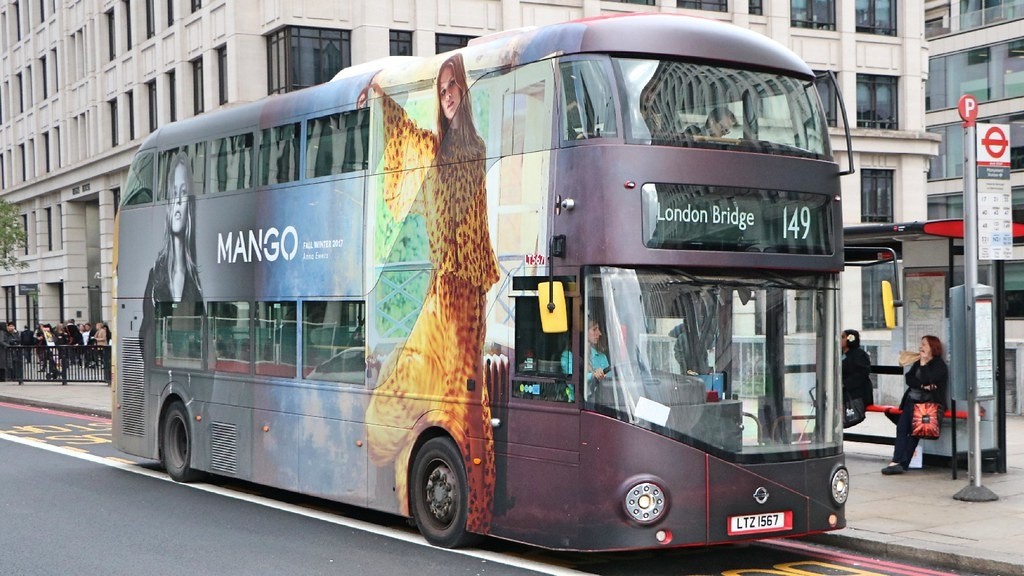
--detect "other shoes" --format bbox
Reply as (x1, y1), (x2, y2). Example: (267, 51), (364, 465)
(38, 368), (46, 372)
(881, 464), (904, 475)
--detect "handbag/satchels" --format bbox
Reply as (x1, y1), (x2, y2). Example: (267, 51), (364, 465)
(69, 337), (77, 345)
(911, 383), (944, 440)
(843, 392), (866, 428)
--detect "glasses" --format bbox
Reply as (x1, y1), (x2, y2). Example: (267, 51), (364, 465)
(718, 122), (731, 134)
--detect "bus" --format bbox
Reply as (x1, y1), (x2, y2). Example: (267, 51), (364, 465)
(109, 10), (906, 556)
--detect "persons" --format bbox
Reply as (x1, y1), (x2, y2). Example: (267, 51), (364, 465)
(136, 152), (217, 472)
(880, 335), (949, 475)
(841, 329), (874, 407)
(560, 319), (612, 404)
(356, 54), (501, 535)
(683, 106), (738, 139)
(667, 319), (708, 378)
(0, 319), (111, 382)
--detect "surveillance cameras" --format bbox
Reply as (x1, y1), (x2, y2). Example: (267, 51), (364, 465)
(95, 272), (99, 280)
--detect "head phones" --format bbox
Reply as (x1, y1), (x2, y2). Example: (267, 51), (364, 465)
(847, 330), (856, 348)
